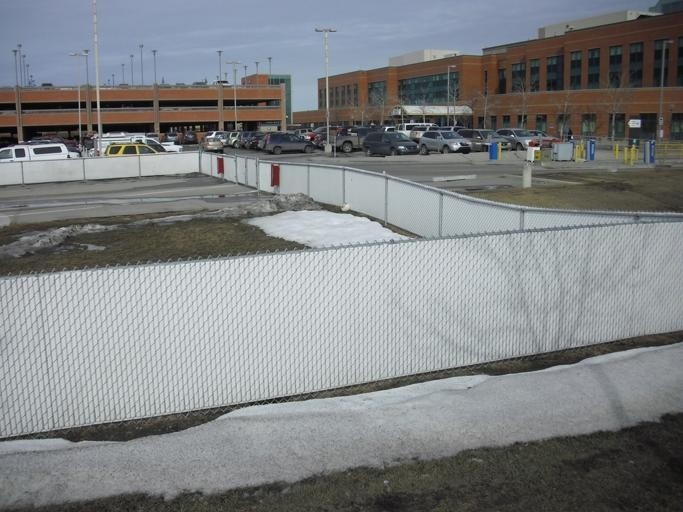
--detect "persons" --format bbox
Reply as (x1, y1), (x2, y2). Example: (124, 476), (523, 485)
(159, 130), (169, 142)
(567, 127), (572, 142)
(195, 128), (204, 150)
(174, 130), (184, 145)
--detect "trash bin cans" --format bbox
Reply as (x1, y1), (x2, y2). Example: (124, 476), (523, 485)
(490, 143), (502, 159)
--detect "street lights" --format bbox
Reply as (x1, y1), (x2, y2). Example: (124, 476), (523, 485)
(11, 43), (35, 125)
(226, 59), (243, 130)
(255, 61), (260, 86)
(447, 65), (456, 125)
(215, 49), (224, 88)
(69, 49), (92, 145)
(315, 28), (338, 153)
(658, 39), (675, 142)
(243, 65), (248, 87)
(268, 57), (272, 85)
(107, 44), (158, 87)
